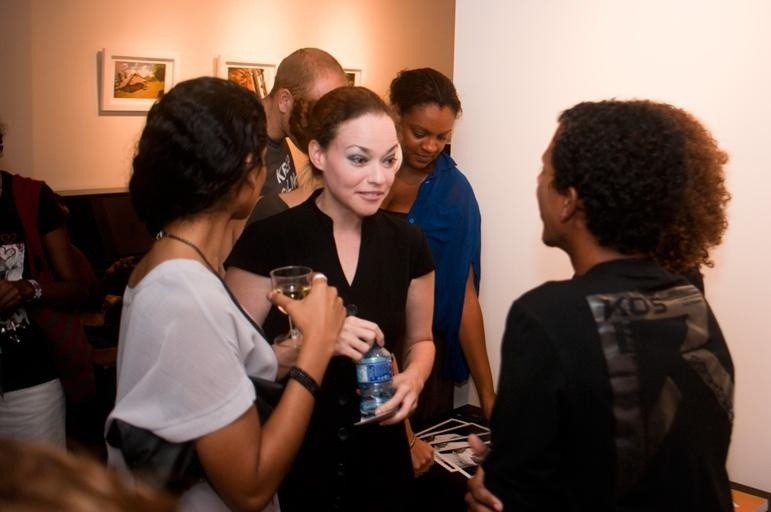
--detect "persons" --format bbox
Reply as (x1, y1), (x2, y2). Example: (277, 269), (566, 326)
(462, 93), (738, 512)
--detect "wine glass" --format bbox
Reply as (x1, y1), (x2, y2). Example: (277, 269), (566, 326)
(268, 264), (315, 350)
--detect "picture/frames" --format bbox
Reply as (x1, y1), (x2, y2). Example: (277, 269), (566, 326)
(99, 48), (174, 112)
(216, 56), (279, 99)
(341, 65), (366, 87)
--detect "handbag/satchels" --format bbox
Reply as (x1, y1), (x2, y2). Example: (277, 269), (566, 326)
(105, 375), (353, 493)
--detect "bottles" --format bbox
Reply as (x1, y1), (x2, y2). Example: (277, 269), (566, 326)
(358, 342), (397, 415)
